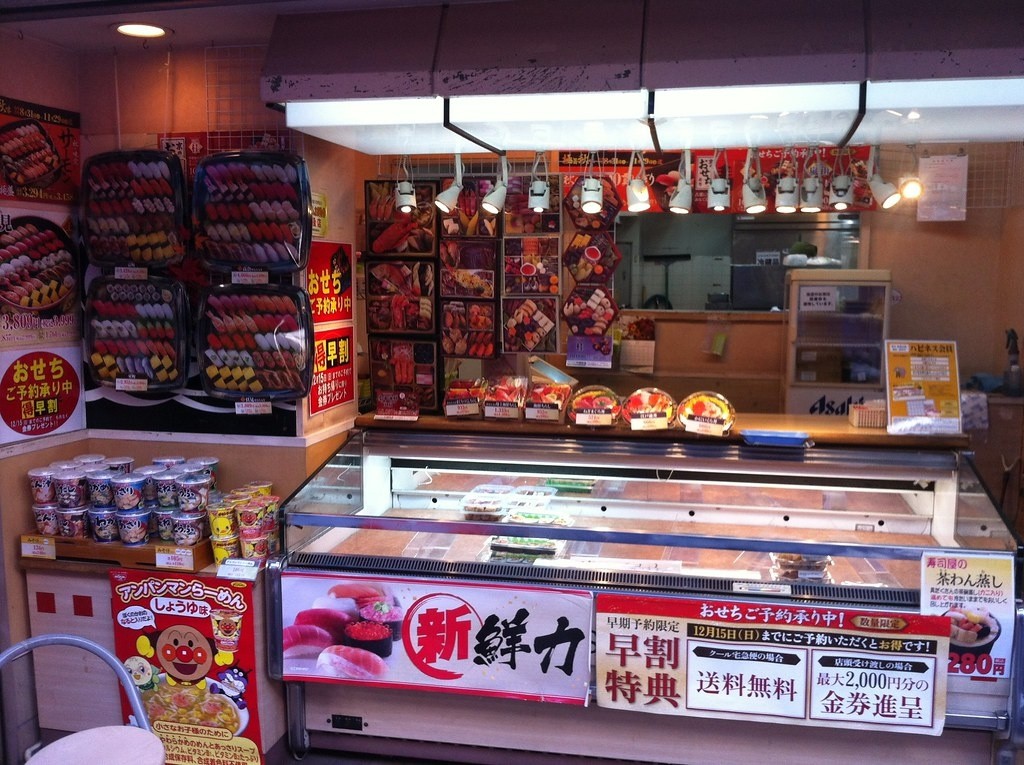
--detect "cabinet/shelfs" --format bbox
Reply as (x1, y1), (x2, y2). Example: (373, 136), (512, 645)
(14, 558), (287, 764)
(959, 388), (1024, 542)
(784, 269), (892, 414)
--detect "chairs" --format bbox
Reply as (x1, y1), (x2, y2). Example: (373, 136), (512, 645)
(0, 634), (165, 765)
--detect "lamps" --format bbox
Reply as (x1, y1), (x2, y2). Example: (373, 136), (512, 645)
(707, 148), (730, 211)
(581, 150), (603, 214)
(528, 150), (549, 213)
(800, 143), (823, 213)
(897, 144), (924, 200)
(669, 149), (692, 214)
(866, 145), (901, 209)
(775, 144), (799, 214)
(481, 155), (509, 215)
(626, 150), (650, 213)
(829, 147), (853, 210)
(434, 153), (465, 214)
(282, 77), (1023, 155)
(395, 155), (416, 213)
(741, 147), (768, 215)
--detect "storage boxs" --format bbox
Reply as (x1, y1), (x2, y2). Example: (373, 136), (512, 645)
(459, 486), (568, 559)
(768, 554), (836, 586)
(544, 478), (595, 495)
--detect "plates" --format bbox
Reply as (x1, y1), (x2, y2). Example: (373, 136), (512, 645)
(365, 175), (619, 411)
(567, 384), (735, 437)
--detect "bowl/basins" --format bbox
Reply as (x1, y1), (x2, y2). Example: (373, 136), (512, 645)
(0, 117), (60, 194)
(941, 610), (1001, 663)
(343, 603), (405, 658)
(0, 215), (75, 316)
